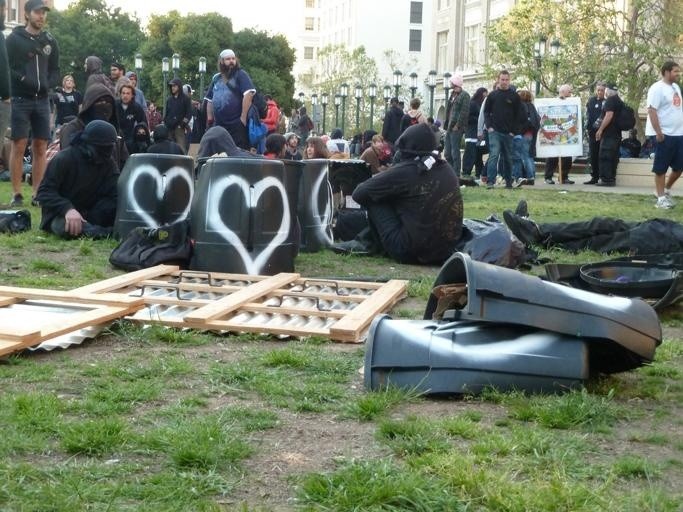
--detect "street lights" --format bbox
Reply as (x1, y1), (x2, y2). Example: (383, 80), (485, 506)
(135, 52), (144, 91)
(367, 82), (377, 133)
(409, 72), (419, 98)
(310, 94), (320, 132)
(319, 93), (329, 136)
(172, 53), (180, 84)
(427, 69), (439, 121)
(161, 56), (172, 120)
(549, 39), (564, 95)
(393, 69), (404, 99)
(532, 36), (546, 95)
(443, 72), (452, 120)
(339, 82), (352, 138)
(382, 85), (393, 117)
(333, 94), (342, 133)
(355, 85), (364, 134)
(197, 56), (208, 101)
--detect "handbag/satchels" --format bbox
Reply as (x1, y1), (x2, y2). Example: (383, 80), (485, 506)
(0, 211), (31, 233)
(334, 210), (368, 239)
(109, 219), (192, 270)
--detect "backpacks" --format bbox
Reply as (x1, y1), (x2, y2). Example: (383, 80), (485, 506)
(617, 101), (635, 131)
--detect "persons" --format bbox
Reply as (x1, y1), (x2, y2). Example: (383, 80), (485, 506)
(503, 199), (683, 268)
(444, 62), (682, 210)
(0, 0), (463, 266)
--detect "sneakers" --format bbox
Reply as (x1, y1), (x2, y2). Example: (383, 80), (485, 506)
(329, 240), (369, 255)
(480, 175), (616, 188)
(515, 200), (528, 216)
(653, 188), (675, 209)
(504, 210), (541, 245)
(11, 193), (24, 208)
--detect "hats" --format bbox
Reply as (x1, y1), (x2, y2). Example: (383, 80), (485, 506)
(607, 83), (617, 90)
(451, 75), (463, 87)
(24, 0), (50, 11)
